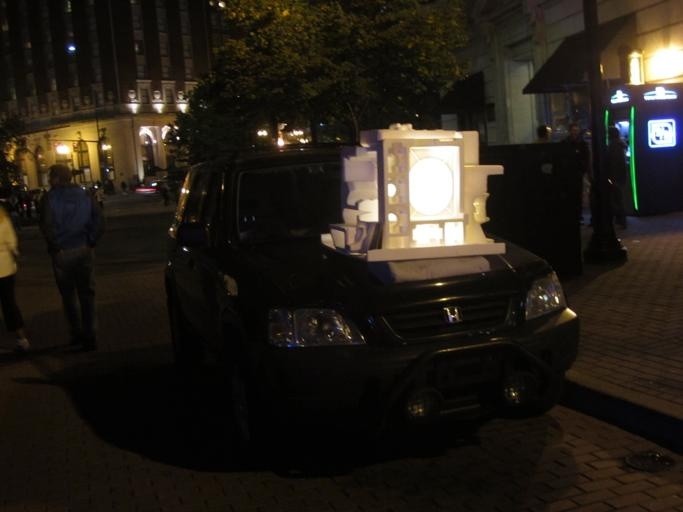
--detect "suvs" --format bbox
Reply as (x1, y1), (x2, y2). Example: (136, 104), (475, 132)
(163, 147), (580, 457)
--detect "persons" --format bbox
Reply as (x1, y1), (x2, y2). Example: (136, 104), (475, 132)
(607, 128), (631, 230)
(562, 120), (591, 170)
(0, 208), (32, 351)
(39, 164), (96, 350)
(159, 180), (172, 206)
(94, 189), (104, 210)
(530, 125), (553, 143)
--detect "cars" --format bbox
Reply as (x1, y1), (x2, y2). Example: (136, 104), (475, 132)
(134, 180), (167, 196)
(21, 188), (48, 212)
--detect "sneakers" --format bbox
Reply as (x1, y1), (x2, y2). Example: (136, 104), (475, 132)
(17, 339), (30, 352)
